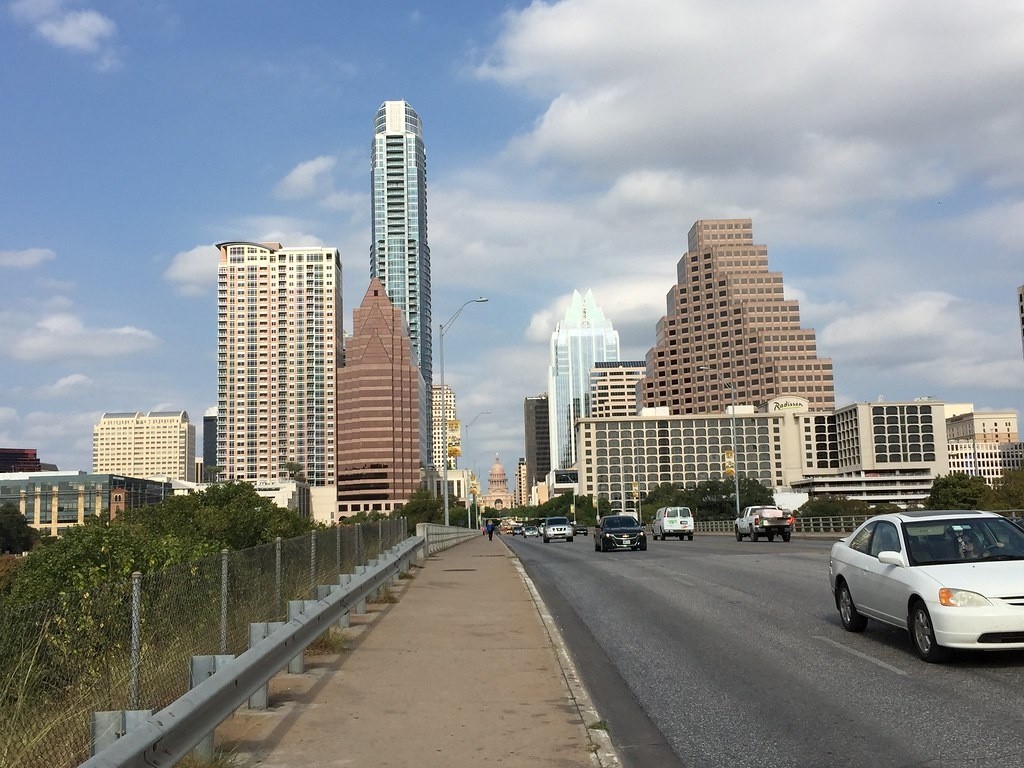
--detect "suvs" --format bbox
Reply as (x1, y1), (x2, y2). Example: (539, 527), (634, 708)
(543, 516), (574, 543)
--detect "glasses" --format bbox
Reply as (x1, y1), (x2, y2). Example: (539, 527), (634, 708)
(964, 539), (973, 544)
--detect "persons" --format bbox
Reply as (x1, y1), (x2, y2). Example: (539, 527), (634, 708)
(955, 535), (1005, 559)
(487, 520), (495, 543)
(481, 526), (485, 535)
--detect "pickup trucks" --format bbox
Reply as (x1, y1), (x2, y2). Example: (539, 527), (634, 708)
(735, 506), (794, 542)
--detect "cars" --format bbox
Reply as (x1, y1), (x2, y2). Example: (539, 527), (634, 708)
(594, 516), (647, 552)
(573, 524), (588, 537)
(829, 510), (1024, 663)
(512, 523), (546, 538)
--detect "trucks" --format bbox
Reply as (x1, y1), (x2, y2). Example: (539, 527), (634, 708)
(650, 506), (695, 541)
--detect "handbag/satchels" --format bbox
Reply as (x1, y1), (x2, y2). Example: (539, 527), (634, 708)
(491, 525), (495, 531)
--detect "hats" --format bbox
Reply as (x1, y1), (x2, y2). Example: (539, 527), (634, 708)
(487, 519), (492, 523)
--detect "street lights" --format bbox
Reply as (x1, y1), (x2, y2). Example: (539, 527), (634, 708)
(466, 410), (492, 530)
(564, 475), (575, 526)
(699, 366), (738, 518)
(439, 297), (489, 526)
